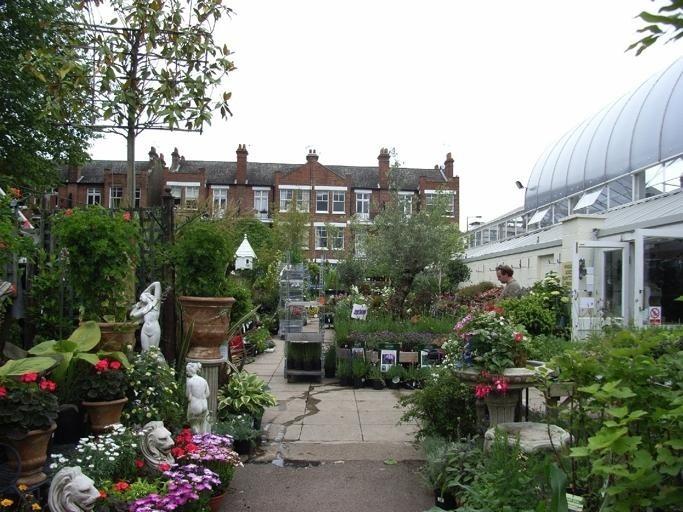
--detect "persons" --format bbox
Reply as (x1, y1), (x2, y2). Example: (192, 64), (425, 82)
(128, 280), (163, 350)
(184, 360), (211, 436)
(493, 264), (522, 304)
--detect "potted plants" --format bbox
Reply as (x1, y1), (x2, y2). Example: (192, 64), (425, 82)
(52, 206), (145, 363)
(214, 370), (277, 455)
(167, 220), (237, 359)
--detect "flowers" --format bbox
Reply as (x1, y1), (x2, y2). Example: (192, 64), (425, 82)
(0, 372), (60, 430)
(126, 345), (182, 426)
(458, 311), (529, 400)
(0, 425), (243, 512)
(77, 358), (125, 399)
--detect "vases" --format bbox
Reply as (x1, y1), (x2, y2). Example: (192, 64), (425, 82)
(79, 400), (127, 431)
